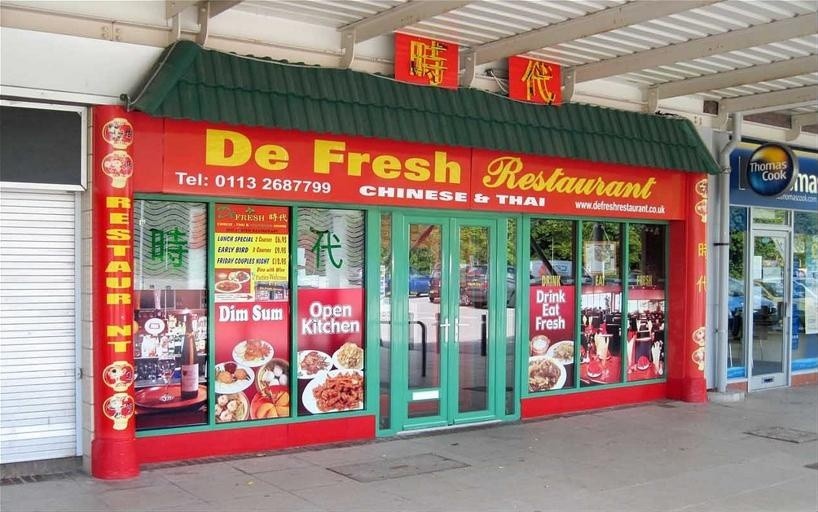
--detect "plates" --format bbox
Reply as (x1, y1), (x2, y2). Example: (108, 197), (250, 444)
(215, 281), (241, 292)
(547, 340), (574, 366)
(332, 347), (364, 370)
(302, 369), (364, 415)
(296, 349), (334, 380)
(249, 385), (290, 420)
(228, 271), (250, 284)
(528, 355), (567, 391)
(233, 339), (275, 367)
(215, 361), (255, 393)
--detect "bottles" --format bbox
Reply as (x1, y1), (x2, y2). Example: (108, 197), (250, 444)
(180, 310), (198, 401)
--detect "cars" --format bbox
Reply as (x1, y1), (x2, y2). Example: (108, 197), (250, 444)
(387, 257), (643, 309)
(728, 277), (817, 336)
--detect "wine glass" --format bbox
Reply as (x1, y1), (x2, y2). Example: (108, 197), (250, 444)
(158, 355), (175, 402)
(594, 335), (610, 376)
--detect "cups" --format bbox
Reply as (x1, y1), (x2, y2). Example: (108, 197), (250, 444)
(652, 345), (662, 375)
